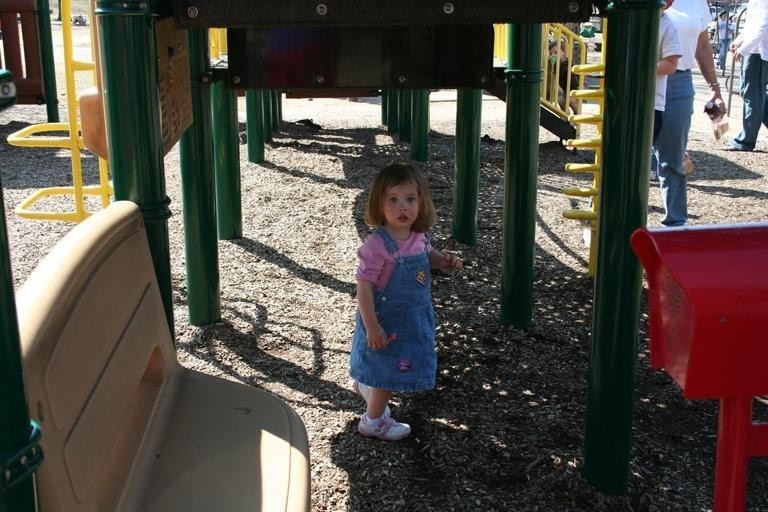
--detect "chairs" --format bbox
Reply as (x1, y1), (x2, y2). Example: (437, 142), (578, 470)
(15, 200), (310, 512)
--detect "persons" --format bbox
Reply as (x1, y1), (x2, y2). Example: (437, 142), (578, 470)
(580, 22), (598, 52)
(348, 162), (465, 444)
(718, 1), (768, 152)
(712, 11), (734, 70)
(654, 0), (728, 228)
(547, 40), (578, 115)
(647, 2), (696, 181)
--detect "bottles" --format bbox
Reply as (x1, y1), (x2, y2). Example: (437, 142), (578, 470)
(704, 100), (722, 123)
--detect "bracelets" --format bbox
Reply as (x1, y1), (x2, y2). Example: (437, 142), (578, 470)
(707, 82), (719, 88)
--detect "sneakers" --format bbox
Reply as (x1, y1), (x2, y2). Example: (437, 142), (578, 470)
(353, 380), (391, 417)
(358, 412), (411, 441)
(582, 228), (592, 248)
(717, 142), (736, 150)
